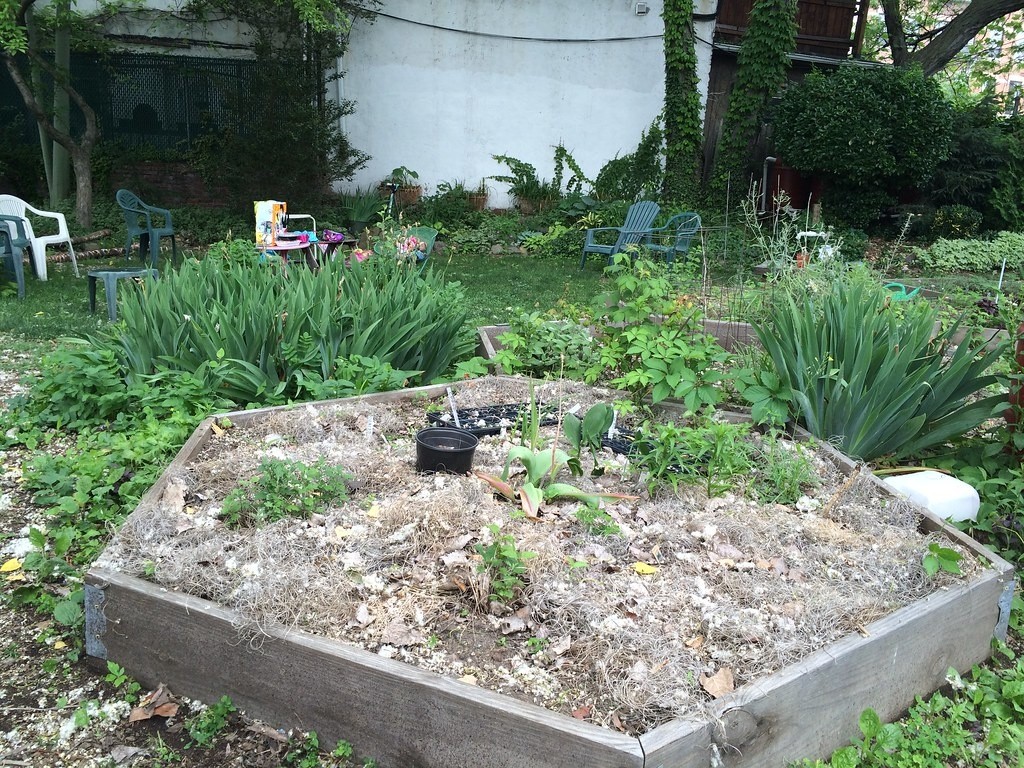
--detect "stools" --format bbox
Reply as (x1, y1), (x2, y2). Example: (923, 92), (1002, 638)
(86, 267), (159, 325)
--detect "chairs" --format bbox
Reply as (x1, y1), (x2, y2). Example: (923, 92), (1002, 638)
(373, 227), (438, 262)
(0, 194), (80, 282)
(116, 189), (177, 269)
(0, 215), (38, 299)
(633, 212), (701, 271)
(580, 200), (660, 276)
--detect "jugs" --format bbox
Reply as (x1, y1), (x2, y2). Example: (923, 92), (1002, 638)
(883, 283), (921, 302)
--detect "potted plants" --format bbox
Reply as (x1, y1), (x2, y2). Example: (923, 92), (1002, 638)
(485, 136), (566, 215)
(379, 166), (422, 207)
(441, 178), (492, 212)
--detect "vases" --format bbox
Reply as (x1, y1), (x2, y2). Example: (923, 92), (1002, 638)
(415, 427), (479, 478)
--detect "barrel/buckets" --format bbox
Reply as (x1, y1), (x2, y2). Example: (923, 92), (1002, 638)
(415, 426), (479, 477)
